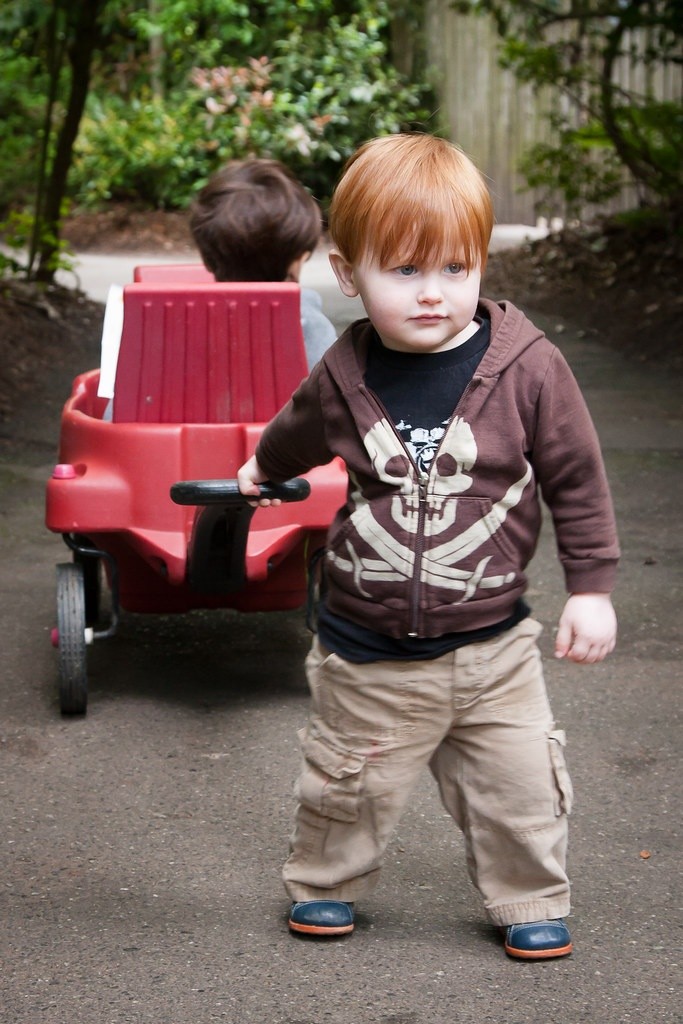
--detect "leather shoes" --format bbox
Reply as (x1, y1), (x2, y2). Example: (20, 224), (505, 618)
(499, 923), (576, 959)
(286, 897), (358, 937)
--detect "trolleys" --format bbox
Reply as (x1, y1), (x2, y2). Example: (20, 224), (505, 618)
(44, 255), (352, 715)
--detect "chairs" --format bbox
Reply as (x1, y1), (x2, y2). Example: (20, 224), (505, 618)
(109, 282), (313, 428)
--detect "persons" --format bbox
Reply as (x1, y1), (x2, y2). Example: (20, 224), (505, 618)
(241, 129), (621, 959)
(96, 158), (339, 424)
